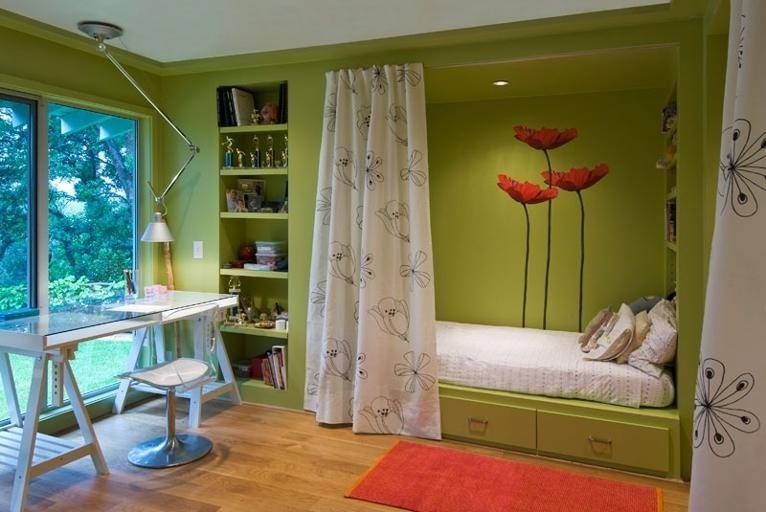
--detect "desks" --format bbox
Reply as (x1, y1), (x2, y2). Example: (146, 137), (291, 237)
(1, 287), (239, 511)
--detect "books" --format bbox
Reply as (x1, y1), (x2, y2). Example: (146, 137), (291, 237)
(261, 345), (286, 390)
(216, 86), (254, 127)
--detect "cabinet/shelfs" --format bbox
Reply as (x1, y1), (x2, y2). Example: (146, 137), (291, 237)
(217, 81), (290, 392)
(661, 80), (679, 314)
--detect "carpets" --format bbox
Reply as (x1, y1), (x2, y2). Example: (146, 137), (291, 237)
(346, 439), (663, 511)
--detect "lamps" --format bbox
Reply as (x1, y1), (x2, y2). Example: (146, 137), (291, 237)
(78, 20), (200, 244)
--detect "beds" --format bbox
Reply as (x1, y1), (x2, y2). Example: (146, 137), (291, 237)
(434, 319), (682, 483)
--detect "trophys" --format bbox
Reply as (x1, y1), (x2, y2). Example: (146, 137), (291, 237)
(251, 134), (260, 169)
(221, 136), (235, 166)
(235, 147), (246, 168)
(265, 133), (274, 169)
(280, 133), (289, 167)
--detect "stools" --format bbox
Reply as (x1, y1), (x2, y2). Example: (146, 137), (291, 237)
(112, 355), (214, 469)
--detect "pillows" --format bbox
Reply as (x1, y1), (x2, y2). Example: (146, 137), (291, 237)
(617, 309), (652, 365)
(585, 302), (635, 361)
(629, 297), (677, 377)
